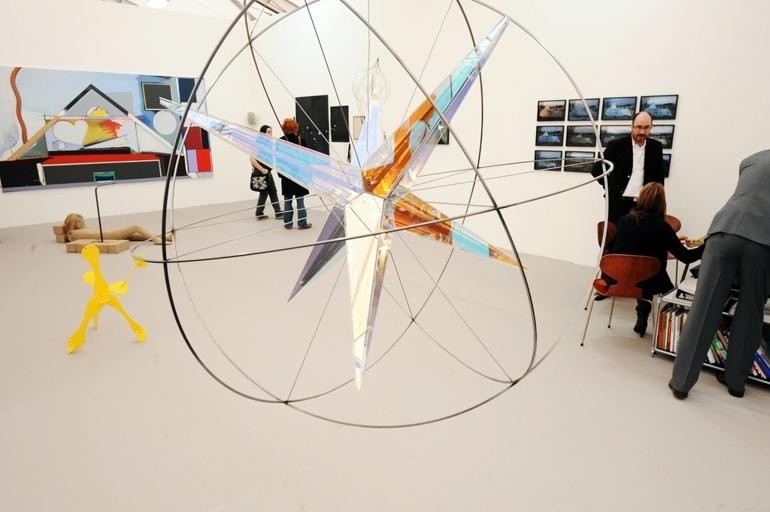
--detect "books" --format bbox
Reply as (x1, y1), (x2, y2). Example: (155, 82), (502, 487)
(657, 303), (770, 380)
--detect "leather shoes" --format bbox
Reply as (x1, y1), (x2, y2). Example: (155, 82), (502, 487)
(716, 370), (744, 397)
(668, 378), (688, 400)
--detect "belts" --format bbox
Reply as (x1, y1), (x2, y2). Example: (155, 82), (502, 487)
(621, 197), (637, 202)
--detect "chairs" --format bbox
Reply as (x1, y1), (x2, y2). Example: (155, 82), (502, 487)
(580, 214), (681, 349)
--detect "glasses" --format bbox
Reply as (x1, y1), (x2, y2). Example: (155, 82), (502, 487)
(633, 124), (652, 131)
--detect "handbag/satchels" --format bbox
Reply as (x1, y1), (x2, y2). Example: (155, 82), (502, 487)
(250, 172), (271, 192)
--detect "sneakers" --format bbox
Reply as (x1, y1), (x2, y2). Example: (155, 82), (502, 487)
(256, 211), (312, 230)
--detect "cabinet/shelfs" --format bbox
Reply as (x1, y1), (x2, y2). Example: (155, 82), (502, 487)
(650, 288), (769, 386)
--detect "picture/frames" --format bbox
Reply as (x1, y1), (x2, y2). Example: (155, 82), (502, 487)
(534, 93), (679, 178)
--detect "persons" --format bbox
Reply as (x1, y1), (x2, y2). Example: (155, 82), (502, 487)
(602, 182), (705, 337)
(274, 117), (312, 230)
(590, 111), (664, 301)
(249, 125), (284, 220)
(667, 148), (770, 400)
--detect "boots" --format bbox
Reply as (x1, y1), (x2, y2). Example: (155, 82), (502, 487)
(634, 291), (653, 338)
